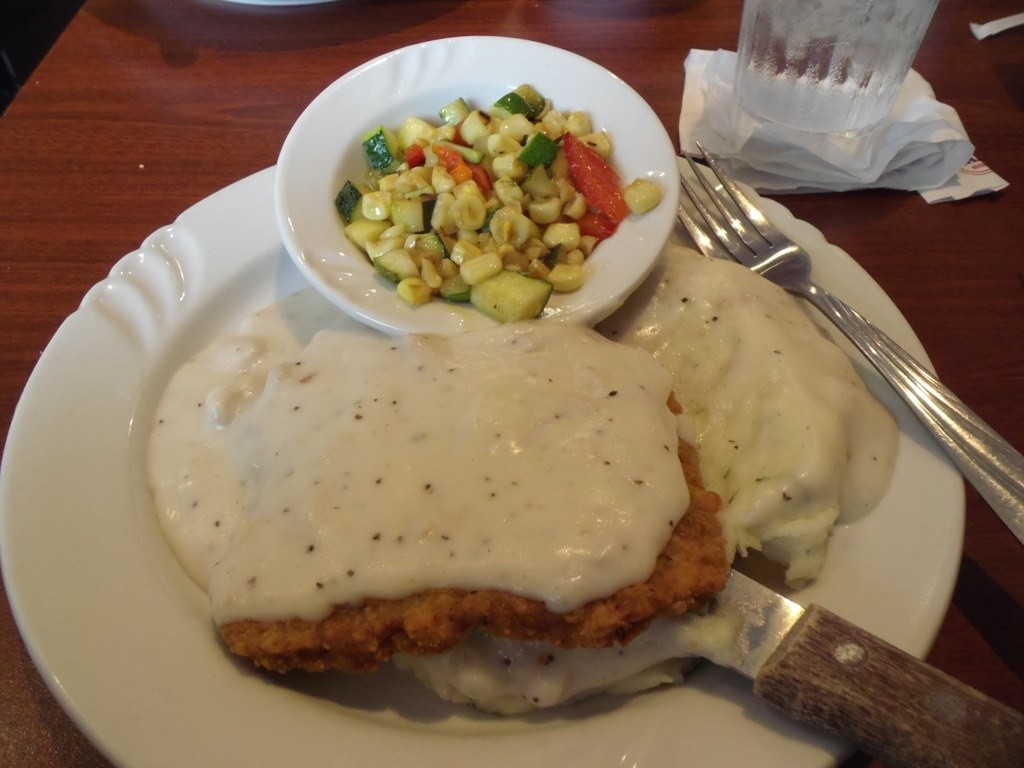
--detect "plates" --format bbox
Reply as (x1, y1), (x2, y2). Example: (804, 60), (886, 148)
(272, 34), (681, 340)
(2, 156), (968, 768)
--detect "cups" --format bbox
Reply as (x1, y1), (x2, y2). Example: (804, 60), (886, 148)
(731, 1), (940, 145)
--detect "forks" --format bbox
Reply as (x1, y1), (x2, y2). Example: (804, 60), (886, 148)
(674, 140), (1023, 554)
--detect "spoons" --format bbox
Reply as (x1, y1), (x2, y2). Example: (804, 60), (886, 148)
(699, 563), (1023, 767)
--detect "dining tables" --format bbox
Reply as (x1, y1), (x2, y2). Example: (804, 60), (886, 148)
(0, 0), (1024, 768)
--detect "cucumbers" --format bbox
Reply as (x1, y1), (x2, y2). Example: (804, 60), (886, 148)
(335, 84), (663, 324)
